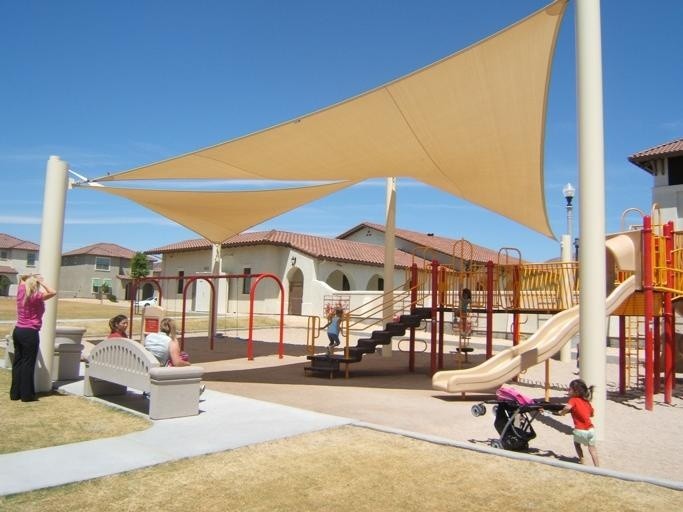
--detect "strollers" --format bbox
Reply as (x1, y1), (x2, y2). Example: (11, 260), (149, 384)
(470, 383), (572, 450)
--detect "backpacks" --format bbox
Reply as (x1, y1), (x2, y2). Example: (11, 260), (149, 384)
(496, 384), (535, 407)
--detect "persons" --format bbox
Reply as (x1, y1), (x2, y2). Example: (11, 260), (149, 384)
(326, 309), (344, 355)
(459, 289), (471, 332)
(144, 318), (205, 395)
(558, 379), (599, 467)
(109, 315), (128, 337)
(10, 274), (56, 402)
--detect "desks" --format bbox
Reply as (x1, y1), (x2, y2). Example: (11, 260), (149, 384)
(53, 326), (86, 380)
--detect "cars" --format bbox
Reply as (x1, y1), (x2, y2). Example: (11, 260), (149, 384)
(133, 295), (157, 308)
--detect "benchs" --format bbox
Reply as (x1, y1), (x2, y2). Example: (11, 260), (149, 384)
(82, 336), (205, 420)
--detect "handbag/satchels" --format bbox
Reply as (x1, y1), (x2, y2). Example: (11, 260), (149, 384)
(166, 351), (189, 367)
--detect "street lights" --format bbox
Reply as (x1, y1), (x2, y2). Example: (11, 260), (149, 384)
(562, 180), (576, 237)
